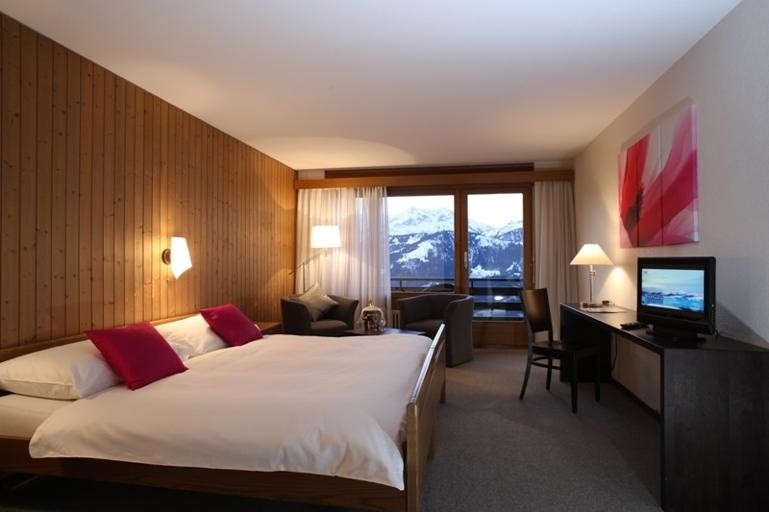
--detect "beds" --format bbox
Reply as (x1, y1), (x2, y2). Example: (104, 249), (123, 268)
(0, 312), (447, 512)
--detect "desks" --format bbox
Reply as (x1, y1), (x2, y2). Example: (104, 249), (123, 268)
(344, 327), (427, 335)
(560, 303), (769, 512)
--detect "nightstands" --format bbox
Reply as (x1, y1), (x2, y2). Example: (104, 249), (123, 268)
(252, 321), (282, 335)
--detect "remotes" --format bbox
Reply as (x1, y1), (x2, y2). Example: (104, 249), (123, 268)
(620, 322), (650, 329)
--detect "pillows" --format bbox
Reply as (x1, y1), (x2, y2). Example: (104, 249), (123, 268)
(154, 313), (228, 359)
(298, 281), (339, 322)
(83, 319), (188, 391)
(0, 338), (195, 400)
(200, 303), (262, 346)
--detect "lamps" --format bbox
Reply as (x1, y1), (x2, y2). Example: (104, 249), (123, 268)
(570, 244), (614, 308)
(162, 236), (193, 279)
(288, 225), (342, 276)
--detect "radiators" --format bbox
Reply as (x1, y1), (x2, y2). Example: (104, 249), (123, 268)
(392, 310), (401, 329)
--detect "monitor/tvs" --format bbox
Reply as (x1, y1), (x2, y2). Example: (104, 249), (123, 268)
(637, 257), (716, 343)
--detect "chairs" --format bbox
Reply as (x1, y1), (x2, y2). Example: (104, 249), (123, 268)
(280, 294), (359, 336)
(397, 294), (475, 368)
(519, 288), (600, 414)
